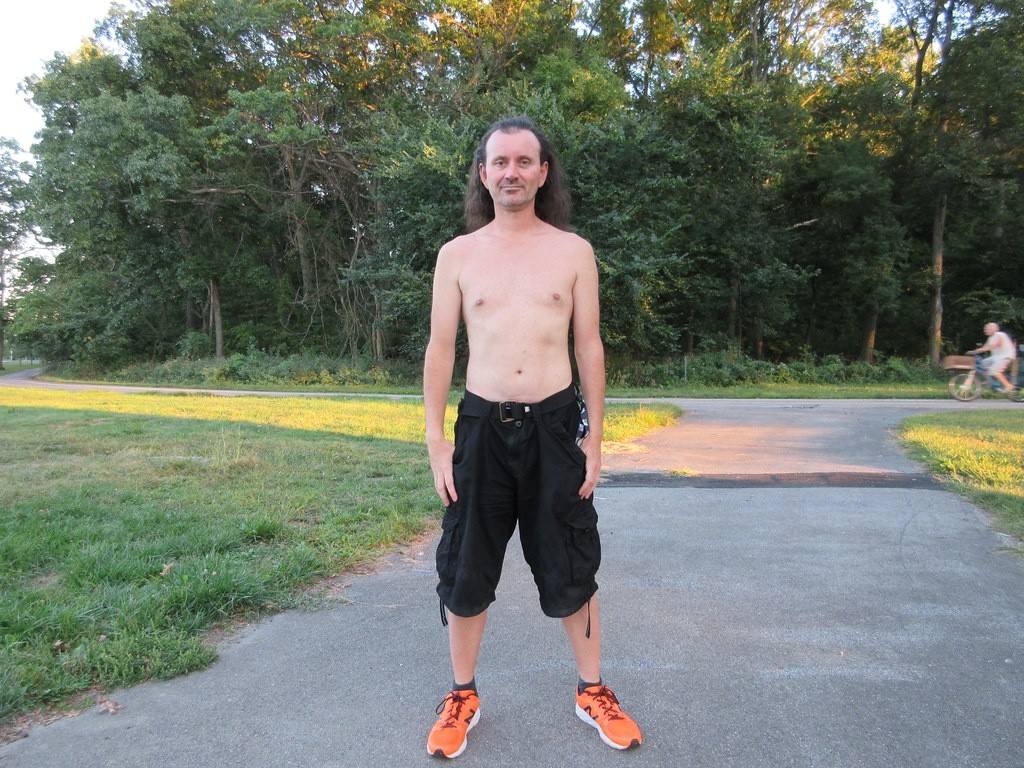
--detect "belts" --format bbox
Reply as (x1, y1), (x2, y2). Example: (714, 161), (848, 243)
(460, 385), (577, 422)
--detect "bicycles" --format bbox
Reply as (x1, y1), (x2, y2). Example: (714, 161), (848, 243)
(942, 351), (1024, 403)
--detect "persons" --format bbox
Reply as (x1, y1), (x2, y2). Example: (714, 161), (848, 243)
(966, 322), (1016, 394)
(421, 117), (647, 760)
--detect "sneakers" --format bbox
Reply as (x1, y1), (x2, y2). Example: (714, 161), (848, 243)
(574, 683), (640, 751)
(426, 689), (480, 758)
